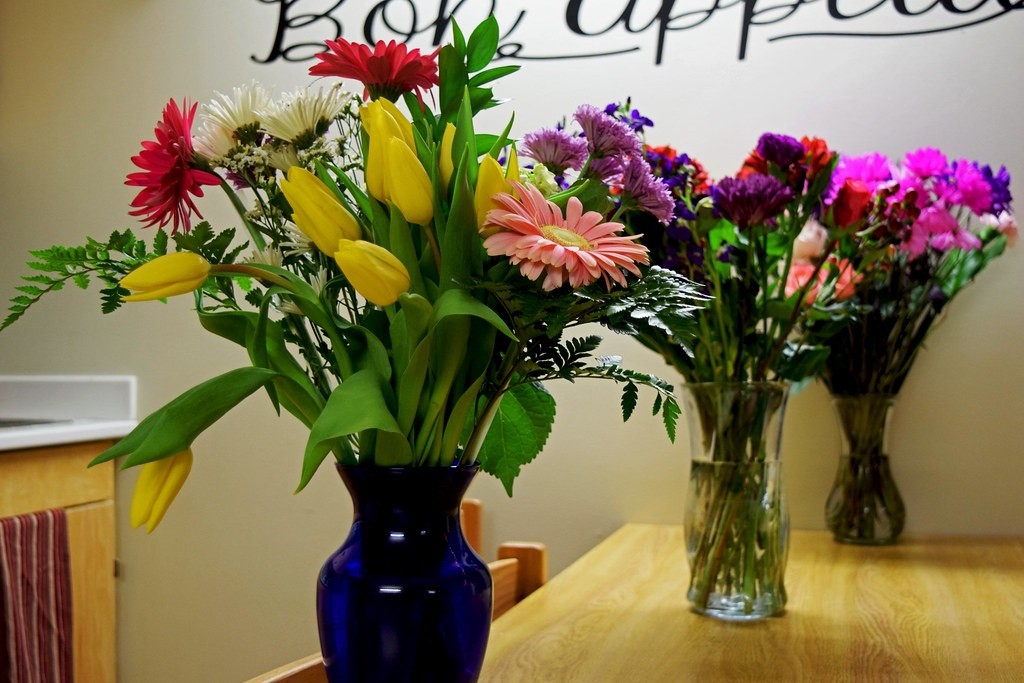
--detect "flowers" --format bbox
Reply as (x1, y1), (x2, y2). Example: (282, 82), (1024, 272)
(0, 15), (714, 534)
(498, 94), (1021, 604)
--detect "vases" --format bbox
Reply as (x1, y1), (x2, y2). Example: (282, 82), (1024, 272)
(676, 381), (789, 621)
(313, 460), (490, 683)
(822, 395), (906, 550)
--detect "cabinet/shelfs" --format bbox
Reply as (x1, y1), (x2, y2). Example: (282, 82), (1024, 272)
(0, 440), (118, 683)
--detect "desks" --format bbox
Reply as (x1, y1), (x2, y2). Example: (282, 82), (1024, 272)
(476, 523), (1023, 683)
